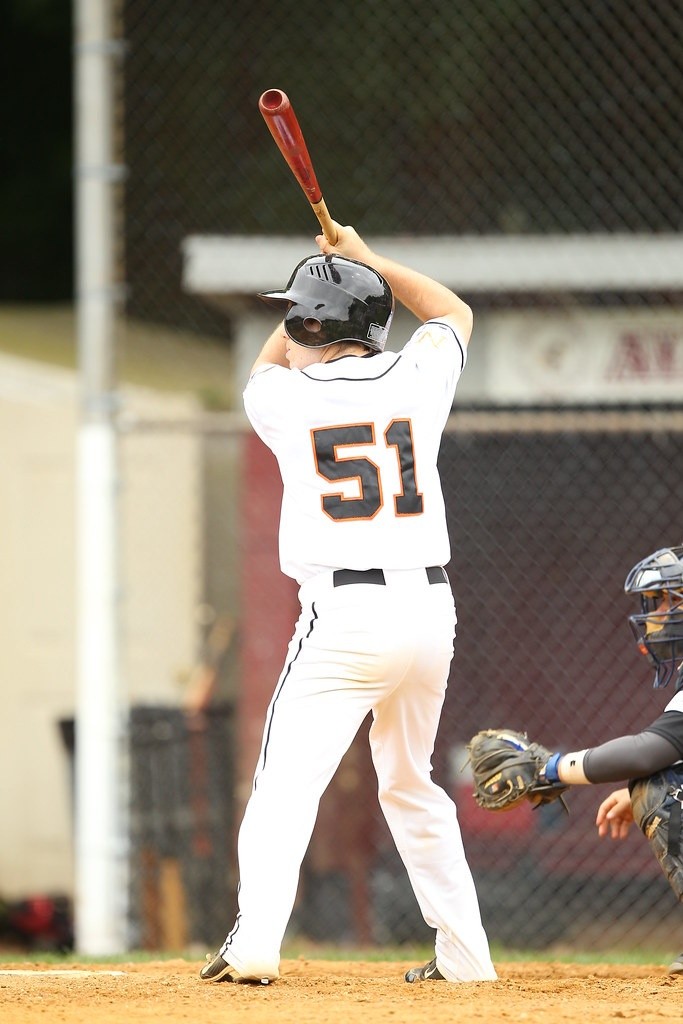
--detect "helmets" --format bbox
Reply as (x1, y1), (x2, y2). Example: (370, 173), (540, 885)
(625, 543), (683, 668)
(255, 253), (395, 353)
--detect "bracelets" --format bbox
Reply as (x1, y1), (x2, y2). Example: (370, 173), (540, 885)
(547, 751), (562, 784)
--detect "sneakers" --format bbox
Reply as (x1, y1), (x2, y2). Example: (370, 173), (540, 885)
(405, 955), (446, 983)
(200, 951), (259, 983)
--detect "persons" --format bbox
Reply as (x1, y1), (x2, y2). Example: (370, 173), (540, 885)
(196, 220), (497, 985)
(525, 547), (683, 976)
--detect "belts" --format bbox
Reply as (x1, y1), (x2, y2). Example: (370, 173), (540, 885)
(333, 566), (446, 587)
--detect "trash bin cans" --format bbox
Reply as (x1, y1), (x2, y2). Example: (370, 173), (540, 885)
(57, 702), (234, 949)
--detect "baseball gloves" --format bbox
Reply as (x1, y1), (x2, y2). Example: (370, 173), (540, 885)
(469, 727), (572, 813)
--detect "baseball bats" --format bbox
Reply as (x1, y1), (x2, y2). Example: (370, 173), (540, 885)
(257, 89), (338, 247)
